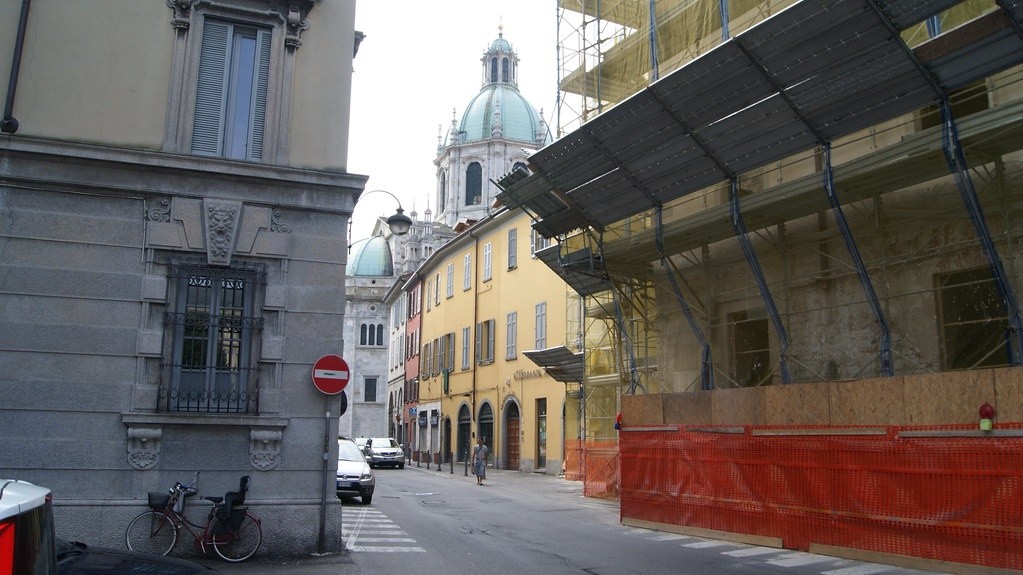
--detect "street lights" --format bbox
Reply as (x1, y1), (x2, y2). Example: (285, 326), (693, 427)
(356, 188), (412, 237)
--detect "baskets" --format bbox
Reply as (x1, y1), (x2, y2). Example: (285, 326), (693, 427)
(148, 491), (170, 509)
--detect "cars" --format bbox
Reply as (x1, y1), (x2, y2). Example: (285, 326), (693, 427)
(351, 437), (372, 453)
(361, 437), (406, 470)
(335, 436), (377, 506)
(53, 529), (225, 575)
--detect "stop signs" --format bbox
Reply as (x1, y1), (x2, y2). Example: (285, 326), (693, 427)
(310, 353), (353, 395)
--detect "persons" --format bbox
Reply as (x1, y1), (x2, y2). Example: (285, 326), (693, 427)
(472, 438), (488, 485)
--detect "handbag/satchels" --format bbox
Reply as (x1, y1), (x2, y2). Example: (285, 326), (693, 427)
(472, 462), (475, 474)
(172, 493), (186, 514)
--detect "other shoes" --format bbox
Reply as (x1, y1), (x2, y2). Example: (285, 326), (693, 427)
(476, 482), (483, 485)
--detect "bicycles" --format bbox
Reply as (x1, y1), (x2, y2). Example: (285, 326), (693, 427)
(123, 473), (266, 563)
